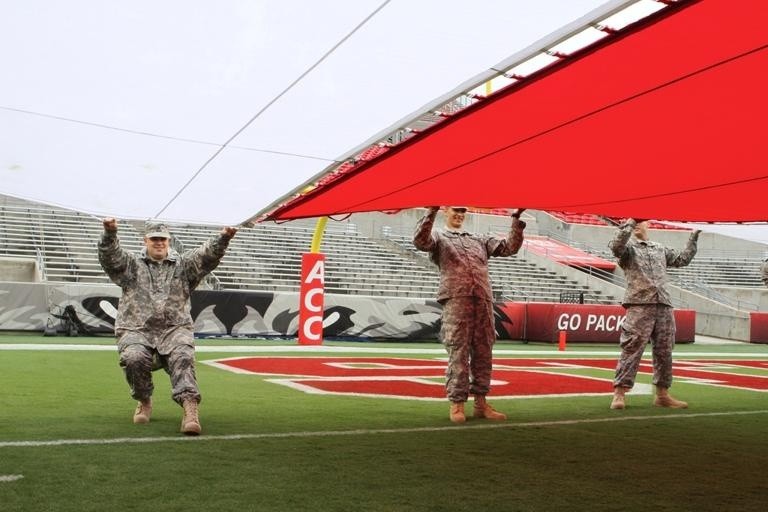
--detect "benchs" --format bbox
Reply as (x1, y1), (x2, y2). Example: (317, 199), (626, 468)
(0, 204), (154, 284)
(156, 221), (452, 300)
(381, 226), (620, 307)
(592, 251), (768, 289)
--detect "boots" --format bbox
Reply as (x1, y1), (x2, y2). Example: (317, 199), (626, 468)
(180, 399), (202, 435)
(473, 395), (507, 421)
(450, 401), (466, 423)
(132, 397), (153, 424)
(609, 386), (625, 409)
(654, 386), (689, 409)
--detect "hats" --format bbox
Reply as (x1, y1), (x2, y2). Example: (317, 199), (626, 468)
(445, 205), (469, 210)
(144, 223), (172, 240)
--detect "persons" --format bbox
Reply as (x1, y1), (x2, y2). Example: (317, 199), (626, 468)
(412, 206), (527, 423)
(96, 218), (240, 436)
(609, 215), (703, 410)
